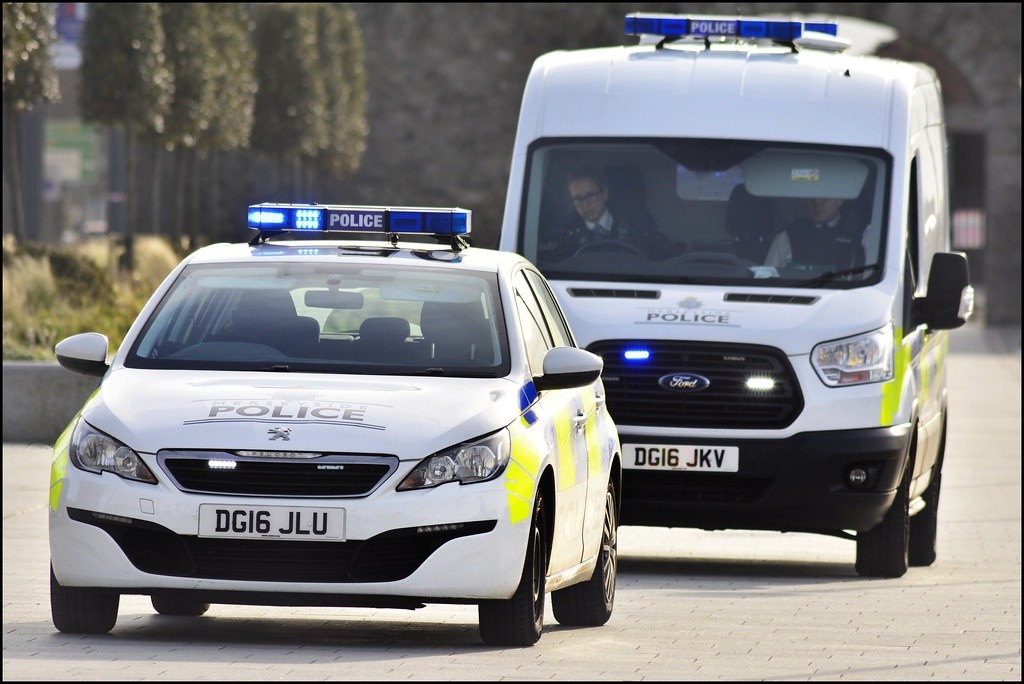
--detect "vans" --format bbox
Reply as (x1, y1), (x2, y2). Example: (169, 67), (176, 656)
(501, 12), (974, 577)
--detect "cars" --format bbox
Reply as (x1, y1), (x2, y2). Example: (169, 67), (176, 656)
(49, 202), (623, 647)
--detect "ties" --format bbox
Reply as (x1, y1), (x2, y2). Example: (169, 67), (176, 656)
(819, 222), (831, 232)
(592, 224), (610, 244)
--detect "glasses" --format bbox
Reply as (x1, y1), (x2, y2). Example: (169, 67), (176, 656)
(571, 190), (605, 204)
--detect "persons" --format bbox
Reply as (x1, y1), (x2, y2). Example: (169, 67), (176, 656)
(548, 168), (670, 265)
(763, 198), (871, 279)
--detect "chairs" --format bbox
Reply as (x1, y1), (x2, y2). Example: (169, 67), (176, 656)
(421, 299), (492, 369)
(215, 285), (320, 360)
(345, 317), (420, 364)
(601, 165), (670, 257)
(721, 181), (778, 262)
